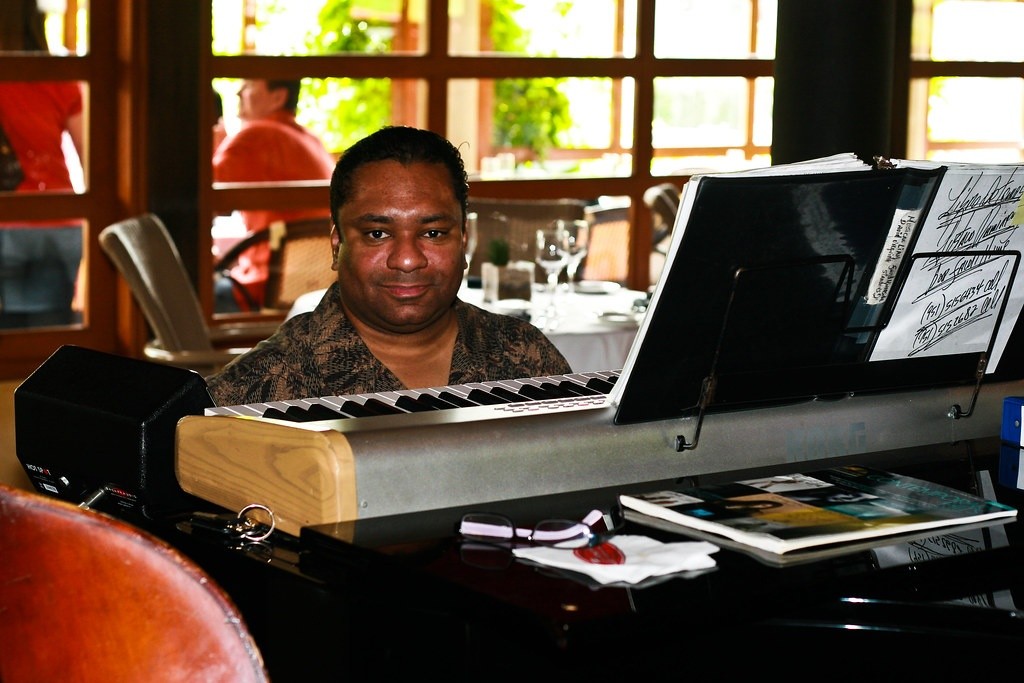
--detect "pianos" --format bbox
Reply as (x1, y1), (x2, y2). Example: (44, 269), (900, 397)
(165, 348), (1024, 553)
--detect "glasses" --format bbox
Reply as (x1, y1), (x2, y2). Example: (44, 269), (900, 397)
(453, 493), (625, 551)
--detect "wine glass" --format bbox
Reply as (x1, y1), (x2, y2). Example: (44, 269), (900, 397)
(534, 228), (571, 298)
(456, 212), (477, 302)
(557, 219), (587, 296)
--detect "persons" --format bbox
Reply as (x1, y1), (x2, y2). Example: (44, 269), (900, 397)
(211, 73), (338, 313)
(204, 125), (593, 408)
(0, 0), (84, 327)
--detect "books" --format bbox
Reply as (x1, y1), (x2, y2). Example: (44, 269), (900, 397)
(606, 462), (1020, 556)
(605, 152), (1024, 426)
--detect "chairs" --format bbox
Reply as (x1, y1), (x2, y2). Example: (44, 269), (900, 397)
(97, 214), (287, 378)
(463, 195), (595, 290)
(0, 482), (270, 683)
(215, 219), (338, 314)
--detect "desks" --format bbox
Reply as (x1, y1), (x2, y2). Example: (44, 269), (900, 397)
(286, 286), (645, 374)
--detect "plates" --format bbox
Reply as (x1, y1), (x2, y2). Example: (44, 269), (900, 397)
(572, 279), (621, 295)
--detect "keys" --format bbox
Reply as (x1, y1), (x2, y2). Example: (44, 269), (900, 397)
(172, 504), (277, 547)
